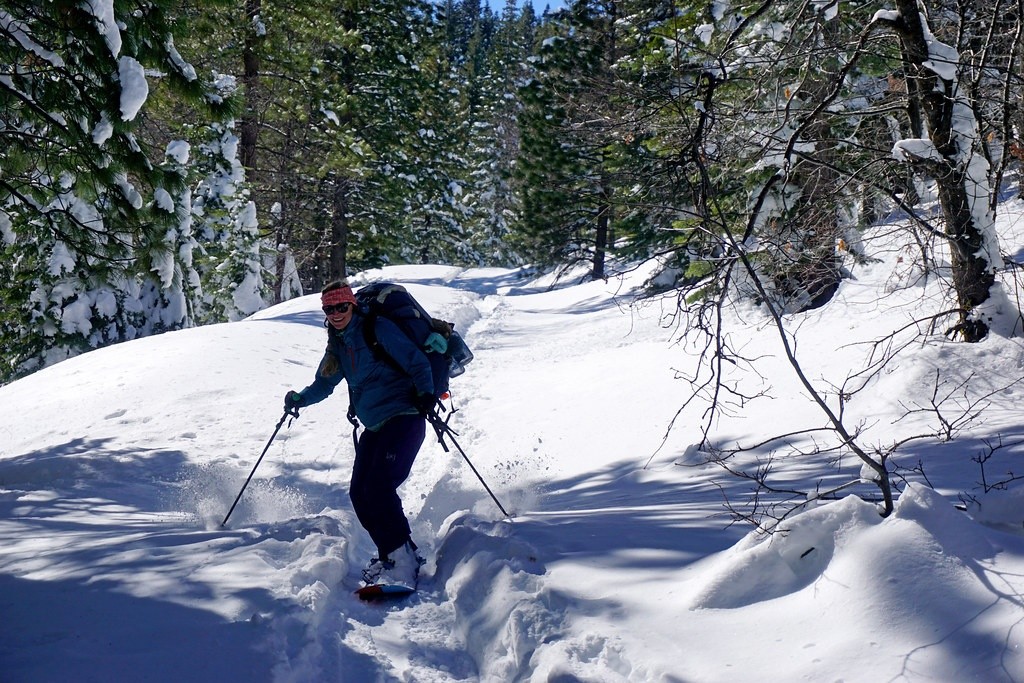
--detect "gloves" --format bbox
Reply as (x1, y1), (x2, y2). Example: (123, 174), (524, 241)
(416, 392), (433, 414)
(285, 391), (305, 412)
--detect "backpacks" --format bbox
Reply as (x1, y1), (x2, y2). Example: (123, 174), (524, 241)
(324, 281), (473, 399)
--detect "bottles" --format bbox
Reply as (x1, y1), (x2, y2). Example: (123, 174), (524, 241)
(448, 329), (474, 366)
(447, 347), (466, 379)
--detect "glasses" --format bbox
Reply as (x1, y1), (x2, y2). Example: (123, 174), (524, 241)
(322, 303), (350, 315)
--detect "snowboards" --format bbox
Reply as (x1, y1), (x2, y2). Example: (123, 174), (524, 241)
(354, 550), (422, 596)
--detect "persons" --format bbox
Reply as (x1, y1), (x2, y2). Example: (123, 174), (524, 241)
(284, 281), (436, 581)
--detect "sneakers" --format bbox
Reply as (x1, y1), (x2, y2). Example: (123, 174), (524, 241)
(377, 541), (419, 586)
(361, 551), (418, 583)
